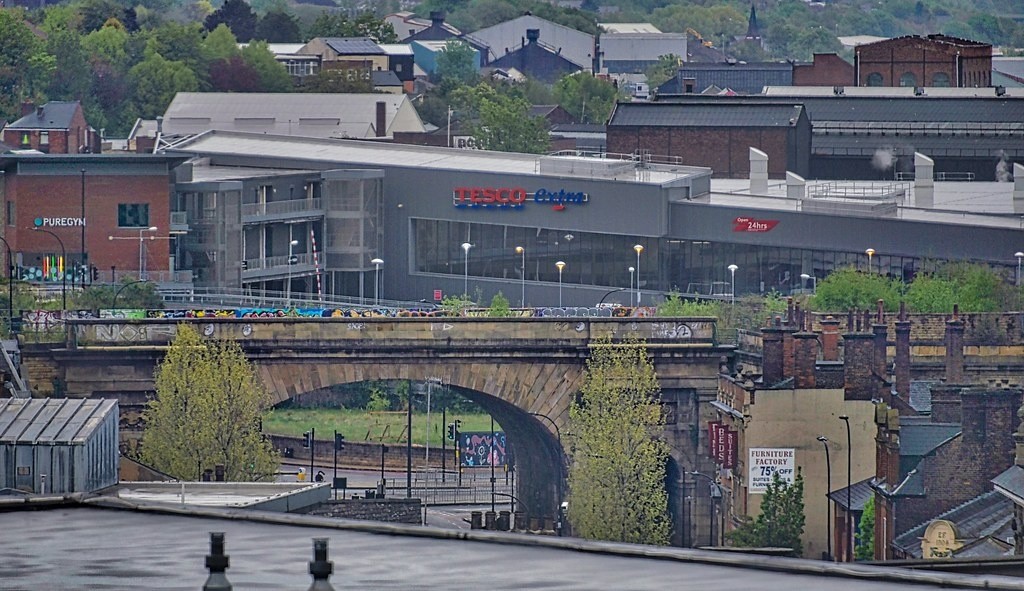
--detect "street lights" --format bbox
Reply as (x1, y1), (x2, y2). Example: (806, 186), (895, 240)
(25, 226), (67, 310)
(489, 492), (530, 533)
(690, 471), (725, 547)
(371, 259), (384, 306)
(586, 453), (626, 545)
(838, 414), (853, 563)
(461, 243), (472, 310)
(516, 246), (525, 309)
(1014, 252), (1024, 285)
(728, 264), (738, 308)
(800, 273), (816, 295)
(815, 435), (833, 558)
(564, 432), (595, 535)
(555, 261), (565, 308)
(138, 226), (158, 281)
(286, 240), (299, 307)
(865, 248), (875, 282)
(528, 411), (562, 536)
(634, 244), (644, 306)
(628, 267), (635, 307)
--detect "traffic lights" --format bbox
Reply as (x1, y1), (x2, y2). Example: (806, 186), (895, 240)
(302, 431), (312, 450)
(447, 423), (456, 441)
